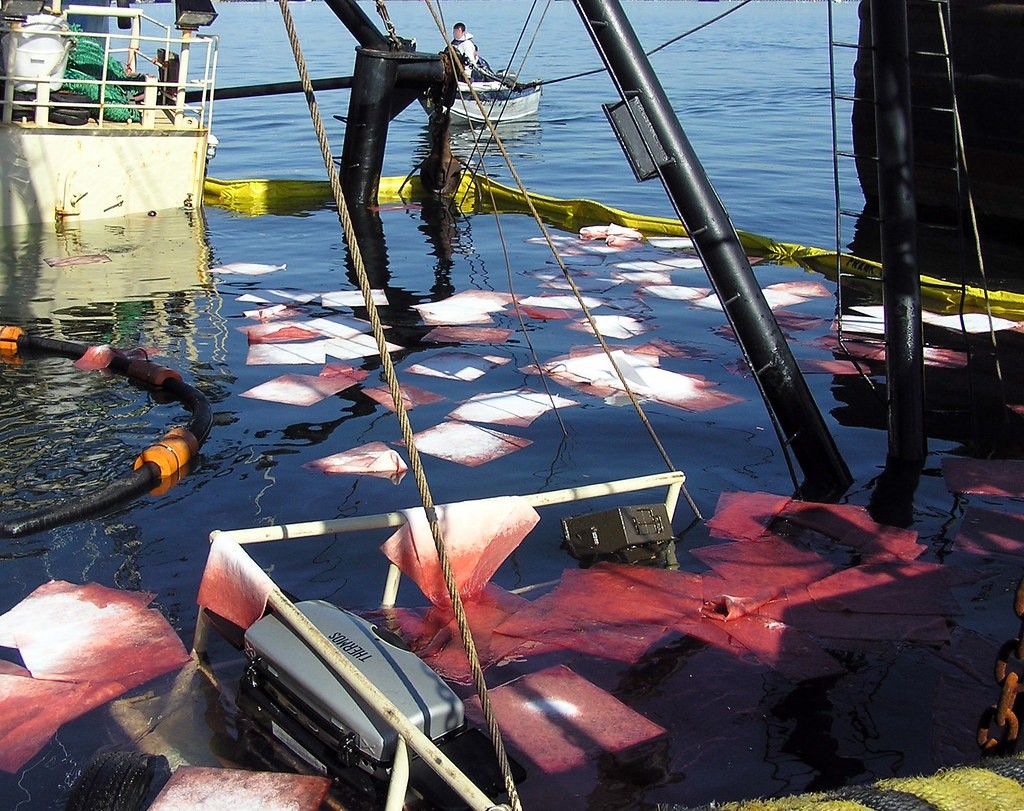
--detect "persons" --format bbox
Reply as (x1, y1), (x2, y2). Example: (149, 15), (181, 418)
(439, 22), (495, 82)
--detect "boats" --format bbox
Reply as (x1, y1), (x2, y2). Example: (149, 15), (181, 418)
(416, 70), (543, 124)
